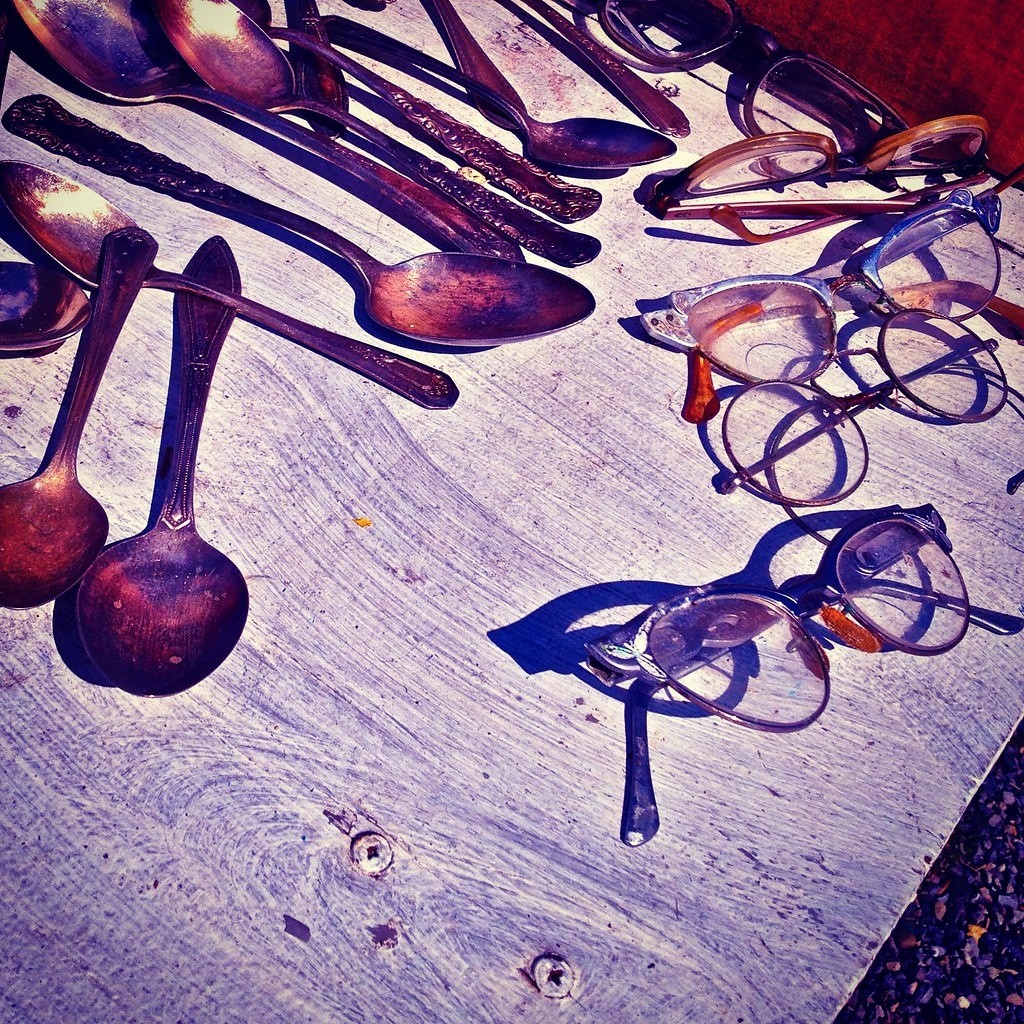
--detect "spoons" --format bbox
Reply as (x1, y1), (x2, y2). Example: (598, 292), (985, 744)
(0, 0), (679, 267)
(0, 160), (459, 410)
(0, 226), (158, 612)
(0, 92), (596, 346)
(74, 234), (251, 698)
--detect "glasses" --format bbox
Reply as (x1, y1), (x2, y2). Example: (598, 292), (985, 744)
(723, 307), (1024, 547)
(643, 114), (1022, 244)
(681, 201), (1023, 422)
(584, 503), (1023, 848)
(596, 0), (912, 189)
